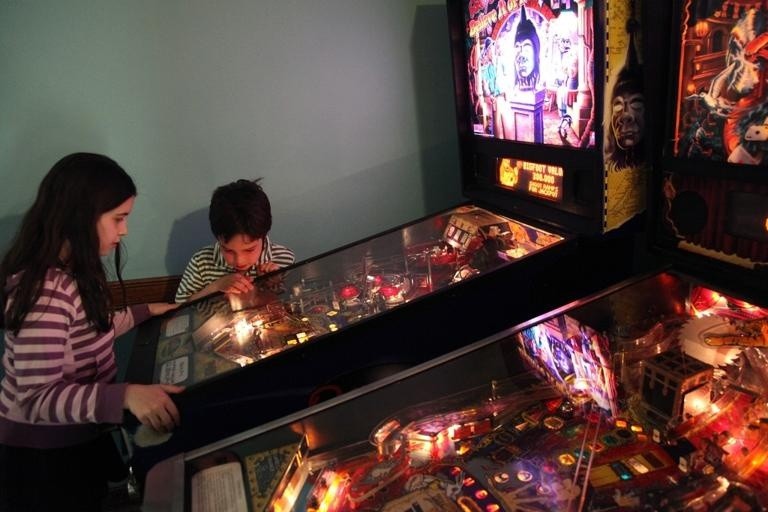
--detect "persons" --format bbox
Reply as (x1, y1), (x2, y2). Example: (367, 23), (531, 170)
(175, 176), (297, 325)
(1, 151), (185, 507)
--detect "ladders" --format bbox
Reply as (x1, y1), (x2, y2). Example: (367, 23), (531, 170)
(567, 368), (612, 512)
